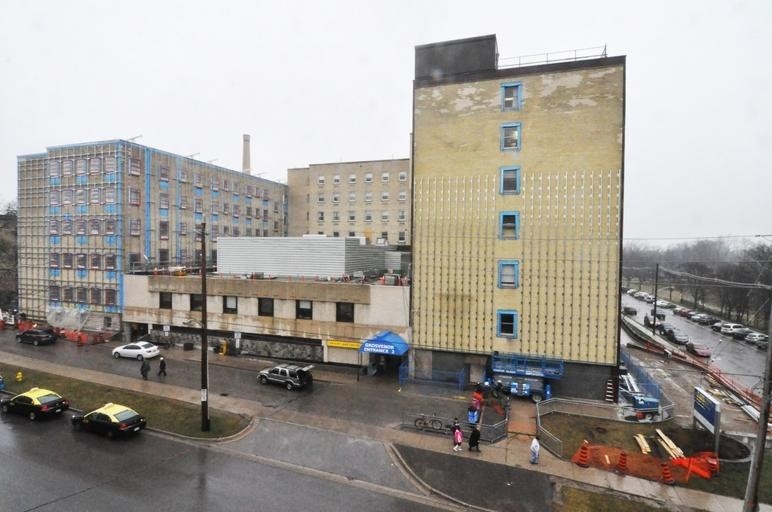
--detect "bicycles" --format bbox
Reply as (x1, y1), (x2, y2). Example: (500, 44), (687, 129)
(414, 412), (443, 431)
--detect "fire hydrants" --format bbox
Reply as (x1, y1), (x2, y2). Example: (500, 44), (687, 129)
(16, 372), (24, 382)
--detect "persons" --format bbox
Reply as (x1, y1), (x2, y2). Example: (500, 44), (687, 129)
(529, 436), (540, 464)
(140, 360), (149, 380)
(450, 380), (502, 452)
(156, 357), (166, 377)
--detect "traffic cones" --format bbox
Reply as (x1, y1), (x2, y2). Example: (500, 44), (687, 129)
(614, 449), (632, 476)
(577, 439), (593, 469)
(658, 460), (674, 484)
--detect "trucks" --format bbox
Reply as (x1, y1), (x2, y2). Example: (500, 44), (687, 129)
(485, 349), (567, 406)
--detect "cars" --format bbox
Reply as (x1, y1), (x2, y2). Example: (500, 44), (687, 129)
(0, 375), (4, 392)
(112, 341), (159, 362)
(0, 387), (69, 420)
(17, 327), (56, 346)
(622, 288), (768, 360)
(71, 403), (148, 439)
(620, 359), (627, 375)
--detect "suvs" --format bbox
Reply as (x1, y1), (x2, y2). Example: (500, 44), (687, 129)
(257, 363), (315, 393)
